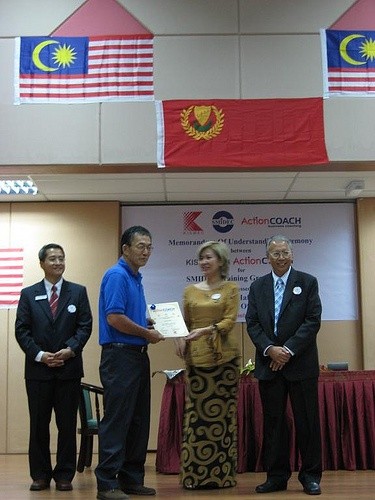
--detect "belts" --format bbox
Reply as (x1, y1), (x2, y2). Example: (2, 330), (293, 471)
(102, 342), (148, 353)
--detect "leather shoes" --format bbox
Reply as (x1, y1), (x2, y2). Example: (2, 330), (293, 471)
(303, 481), (322, 495)
(55, 478), (73, 491)
(256, 479), (287, 493)
(96, 488), (130, 500)
(120, 484), (156, 495)
(30, 478), (51, 491)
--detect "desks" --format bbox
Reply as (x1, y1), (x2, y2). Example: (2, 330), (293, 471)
(155, 370), (375, 475)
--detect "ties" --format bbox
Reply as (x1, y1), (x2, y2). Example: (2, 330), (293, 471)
(274, 277), (285, 337)
(48, 285), (60, 318)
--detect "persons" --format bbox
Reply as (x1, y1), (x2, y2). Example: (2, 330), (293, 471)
(245, 236), (323, 494)
(93, 225), (166, 500)
(175, 241), (242, 490)
(14, 243), (93, 490)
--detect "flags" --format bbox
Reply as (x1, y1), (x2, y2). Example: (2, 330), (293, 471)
(155, 97), (329, 169)
(14, 34), (154, 105)
(320, 29), (375, 98)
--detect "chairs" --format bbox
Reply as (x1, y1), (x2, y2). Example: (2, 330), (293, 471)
(76, 383), (104, 474)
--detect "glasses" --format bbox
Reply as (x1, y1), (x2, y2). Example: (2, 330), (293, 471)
(268, 250), (293, 261)
(136, 245), (155, 253)
